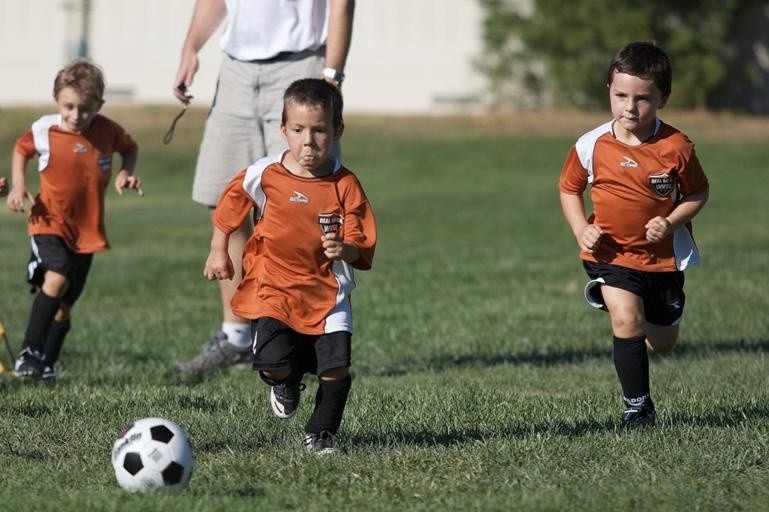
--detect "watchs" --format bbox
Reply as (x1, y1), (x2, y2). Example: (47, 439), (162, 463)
(321, 67), (345, 86)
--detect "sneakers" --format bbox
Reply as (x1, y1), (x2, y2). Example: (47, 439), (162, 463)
(270, 375), (307, 419)
(43, 365), (56, 380)
(305, 431), (339, 457)
(178, 329), (253, 378)
(12, 347), (48, 380)
(619, 399), (657, 434)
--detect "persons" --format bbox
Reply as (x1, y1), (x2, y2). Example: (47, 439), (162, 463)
(4, 61), (147, 386)
(164, 1), (354, 383)
(200, 79), (377, 460)
(558, 41), (711, 433)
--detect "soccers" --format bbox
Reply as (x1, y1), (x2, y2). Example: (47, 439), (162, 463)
(111, 417), (193, 493)
